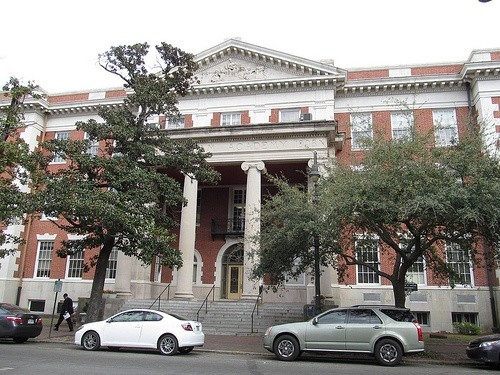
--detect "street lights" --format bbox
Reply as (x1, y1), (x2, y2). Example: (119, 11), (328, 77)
(310, 151), (321, 316)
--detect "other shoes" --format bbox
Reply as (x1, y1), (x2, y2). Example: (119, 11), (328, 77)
(69, 329), (74, 332)
(53, 327), (58, 331)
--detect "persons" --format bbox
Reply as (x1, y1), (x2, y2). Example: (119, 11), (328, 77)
(53, 293), (74, 332)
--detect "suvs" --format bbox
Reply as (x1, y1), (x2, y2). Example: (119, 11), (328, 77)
(262, 304), (424, 367)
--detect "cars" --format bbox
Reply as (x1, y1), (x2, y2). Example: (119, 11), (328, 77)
(75, 308), (204, 356)
(0, 303), (42, 344)
(467, 333), (500, 368)
